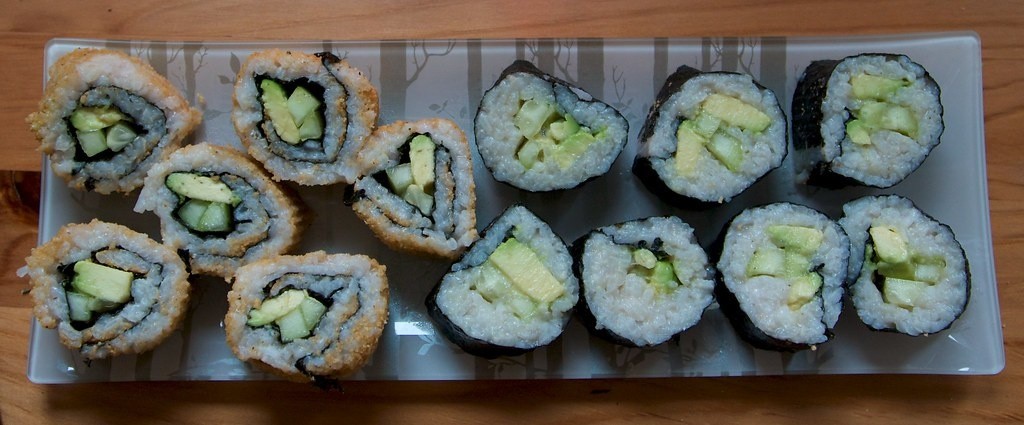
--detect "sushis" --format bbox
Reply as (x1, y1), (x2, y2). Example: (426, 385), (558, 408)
(426, 54), (972, 355)
(17, 49), (476, 378)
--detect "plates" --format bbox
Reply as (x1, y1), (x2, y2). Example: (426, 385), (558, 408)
(26, 31), (1004, 382)
